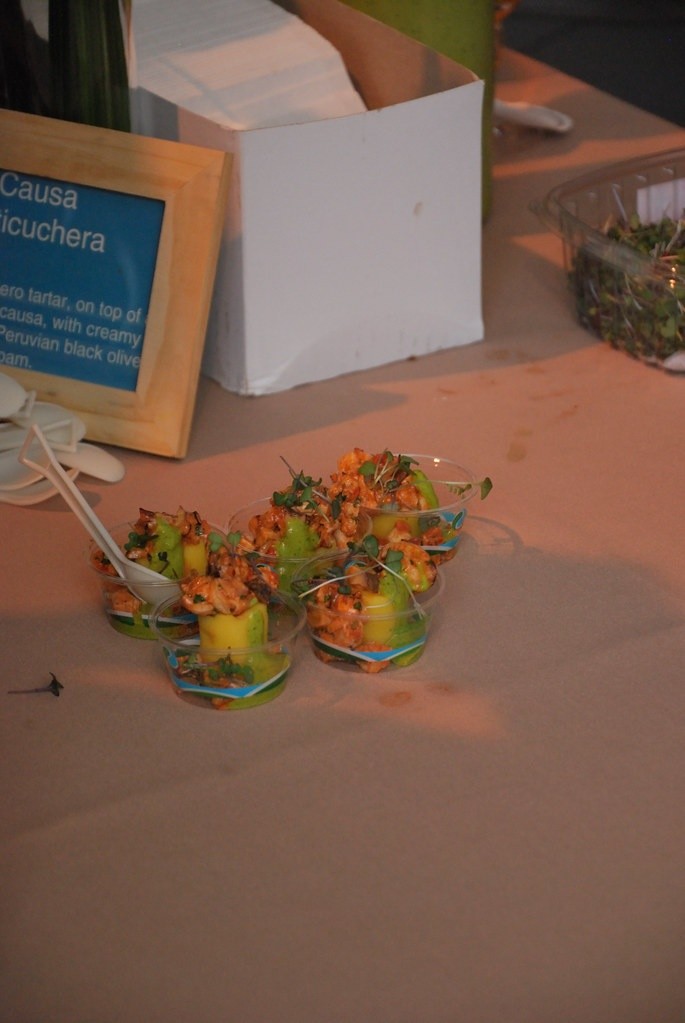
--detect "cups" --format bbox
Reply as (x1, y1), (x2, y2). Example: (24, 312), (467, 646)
(86, 522), (233, 641)
(289, 547), (446, 675)
(147, 583), (308, 711)
(366, 454), (481, 565)
(224, 496), (374, 614)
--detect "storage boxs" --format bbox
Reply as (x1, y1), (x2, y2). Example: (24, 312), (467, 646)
(138, 0), (484, 395)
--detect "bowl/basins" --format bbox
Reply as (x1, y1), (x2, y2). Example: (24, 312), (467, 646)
(526, 147), (685, 373)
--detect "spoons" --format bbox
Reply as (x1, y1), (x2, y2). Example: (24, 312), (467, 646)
(0, 372), (124, 507)
(18, 424), (180, 605)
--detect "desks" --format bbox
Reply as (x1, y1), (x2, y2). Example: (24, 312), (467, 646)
(0, 44), (685, 1023)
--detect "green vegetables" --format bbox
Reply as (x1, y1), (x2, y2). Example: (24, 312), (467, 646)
(99, 449), (494, 688)
(568, 210), (685, 363)
